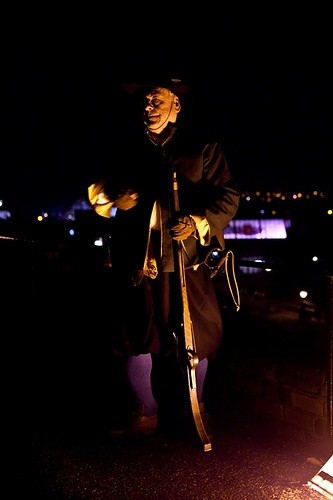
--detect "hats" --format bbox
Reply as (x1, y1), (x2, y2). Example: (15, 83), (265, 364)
(120, 73), (190, 100)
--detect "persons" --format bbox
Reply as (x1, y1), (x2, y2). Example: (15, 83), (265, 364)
(81, 74), (240, 445)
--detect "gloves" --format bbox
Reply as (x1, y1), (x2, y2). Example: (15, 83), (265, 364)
(170, 216), (195, 242)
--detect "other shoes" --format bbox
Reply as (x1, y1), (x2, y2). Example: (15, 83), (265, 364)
(131, 406), (161, 441)
(183, 401), (213, 442)
